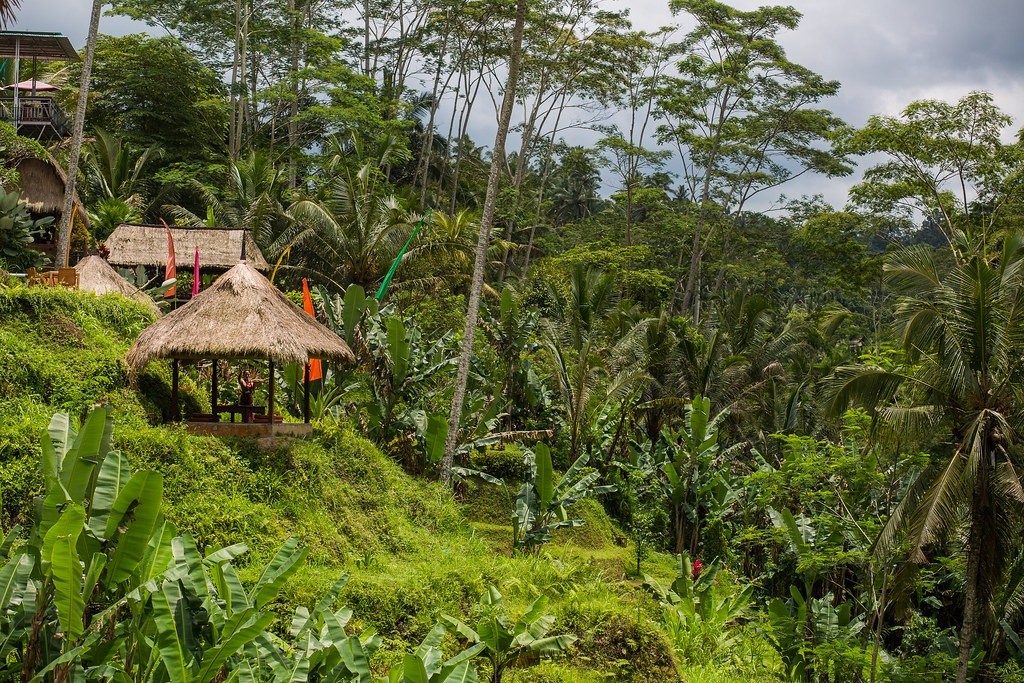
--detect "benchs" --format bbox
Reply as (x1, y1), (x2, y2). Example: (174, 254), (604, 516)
(191, 413), (221, 423)
(253, 415), (283, 424)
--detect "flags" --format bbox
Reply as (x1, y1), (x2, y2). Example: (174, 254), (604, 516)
(371, 217), (425, 301)
(192, 245), (200, 298)
(159, 217), (176, 298)
(299, 276), (326, 384)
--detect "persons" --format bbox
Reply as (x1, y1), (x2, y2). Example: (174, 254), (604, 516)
(237, 366), (264, 423)
(691, 559), (703, 581)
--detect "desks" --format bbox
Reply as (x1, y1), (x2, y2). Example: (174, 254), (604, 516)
(211, 405), (266, 423)
